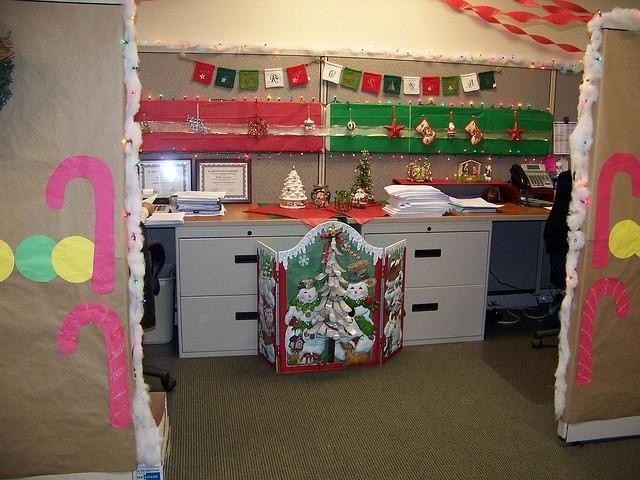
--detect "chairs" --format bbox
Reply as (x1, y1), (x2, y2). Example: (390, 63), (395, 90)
(139, 218), (178, 394)
(531, 167), (574, 352)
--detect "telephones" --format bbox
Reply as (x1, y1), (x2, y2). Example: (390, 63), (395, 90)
(510, 163), (554, 189)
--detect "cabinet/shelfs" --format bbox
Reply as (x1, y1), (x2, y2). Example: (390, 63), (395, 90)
(174, 214), (337, 361)
(347, 211), (493, 350)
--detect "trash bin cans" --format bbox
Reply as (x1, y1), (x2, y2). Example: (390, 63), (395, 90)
(143, 276), (173, 345)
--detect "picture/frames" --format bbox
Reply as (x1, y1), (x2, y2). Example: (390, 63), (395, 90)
(195, 158), (254, 205)
(139, 157), (193, 205)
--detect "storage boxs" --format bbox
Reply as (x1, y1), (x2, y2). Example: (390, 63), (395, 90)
(131, 389), (174, 480)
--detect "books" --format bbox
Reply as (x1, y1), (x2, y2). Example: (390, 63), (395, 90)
(382, 185), (505, 217)
(145, 191), (227, 226)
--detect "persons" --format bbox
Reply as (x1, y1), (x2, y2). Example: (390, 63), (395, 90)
(140, 202), (156, 222)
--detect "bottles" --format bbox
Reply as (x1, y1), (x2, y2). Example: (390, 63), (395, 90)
(551, 161), (564, 198)
